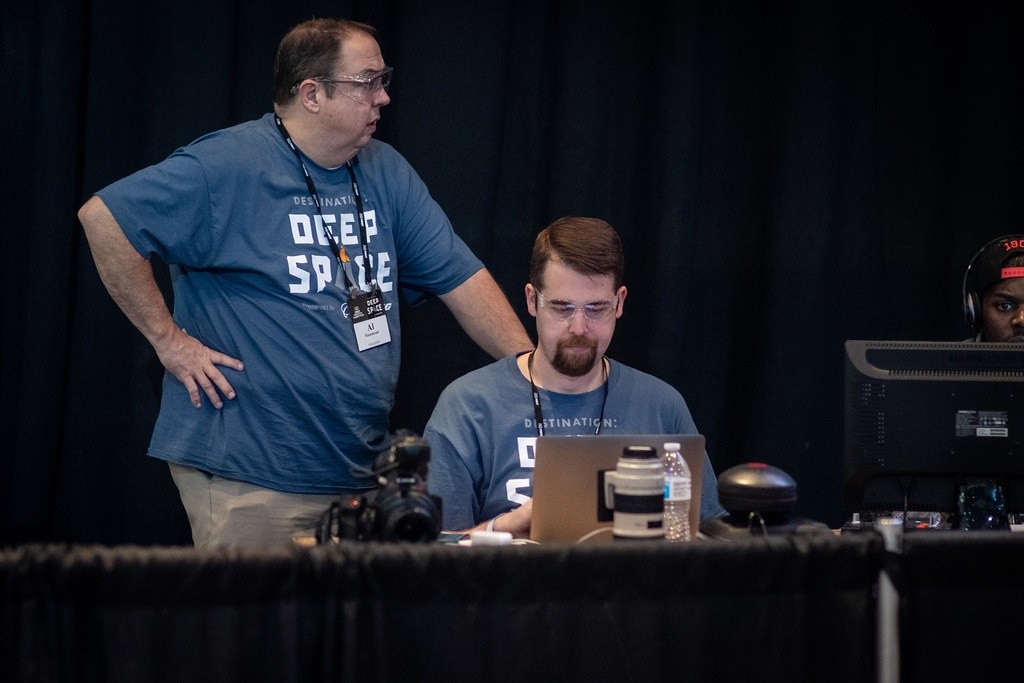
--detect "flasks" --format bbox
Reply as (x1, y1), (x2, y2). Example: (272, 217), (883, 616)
(605, 445), (666, 543)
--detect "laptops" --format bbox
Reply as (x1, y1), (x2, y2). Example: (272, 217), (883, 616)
(528, 433), (705, 546)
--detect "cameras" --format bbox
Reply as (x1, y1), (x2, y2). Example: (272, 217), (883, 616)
(315, 427), (443, 544)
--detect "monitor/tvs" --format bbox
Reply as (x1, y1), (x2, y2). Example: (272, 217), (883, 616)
(840, 340), (1023, 482)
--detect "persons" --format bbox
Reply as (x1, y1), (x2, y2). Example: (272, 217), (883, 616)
(74, 18), (537, 554)
(956, 234), (1024, 343)
(419, 215), (727, 540)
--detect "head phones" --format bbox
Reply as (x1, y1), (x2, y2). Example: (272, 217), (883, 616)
(961, 234), (1024, 335)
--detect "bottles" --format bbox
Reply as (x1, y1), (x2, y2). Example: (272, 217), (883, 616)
(661, 441), (692, 544)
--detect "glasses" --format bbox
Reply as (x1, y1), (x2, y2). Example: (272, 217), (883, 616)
(291, 66), (395, 105)
(534, 283), (621, 323)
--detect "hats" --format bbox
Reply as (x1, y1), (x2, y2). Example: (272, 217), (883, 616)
(968, 236), (1024, 298)
